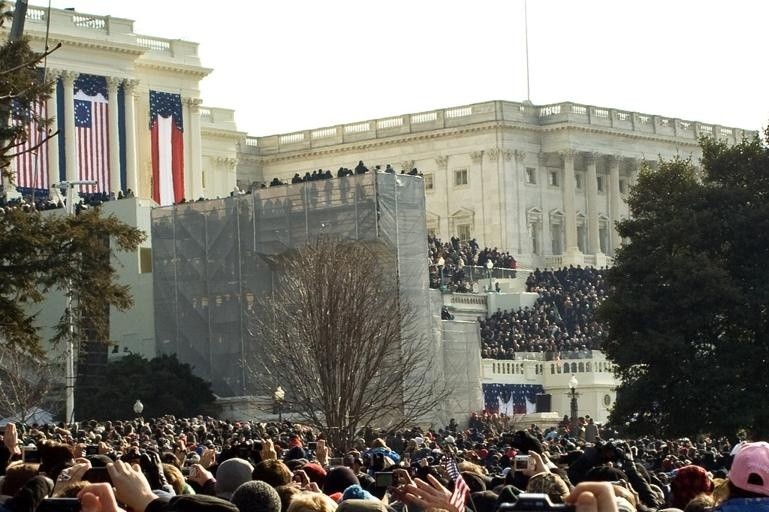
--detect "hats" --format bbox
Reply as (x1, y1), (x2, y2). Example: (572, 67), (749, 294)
(727, 442), (769, 495)
(337, 485), (378, 500)
(216, 458), (254, 500)
(335, 499), (388, 512)
(303, 463), (326, 485)
(676, 466), (715, 500)
(528, 471), (569, 495)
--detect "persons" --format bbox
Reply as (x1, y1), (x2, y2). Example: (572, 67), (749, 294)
(0, 412), (769, 512)
(246, 160), (369, 195)
(179, 197), (209, 204)
(427, 233), (616, 368)
(376, 164), (423, 176)
(0, 183), (134, 216)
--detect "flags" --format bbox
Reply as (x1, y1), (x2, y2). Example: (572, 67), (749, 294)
(12, 67), (127, 199)
(149, 89), (184, 206)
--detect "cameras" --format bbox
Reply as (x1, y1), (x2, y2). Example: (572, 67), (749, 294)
(22, 446), (43, 465)
(36, 497), (81, 512)
(180, 467), (197, 478)
(374, 472), (398, 488)
(82, 467), (112, 483)
(252, 440), (263, 452)
(514, 454), (535, 472)
(307, 441), (318, 450)
(86, 446), (99, 455)
(498, 492), (576, 512)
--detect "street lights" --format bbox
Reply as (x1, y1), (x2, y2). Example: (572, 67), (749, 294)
(273, 386), (286, 421)
(565, 374), (582, 421)
(437, 257), (450, 293)
(132, 399), (144, 417)
(486, 260), (495, 292)
